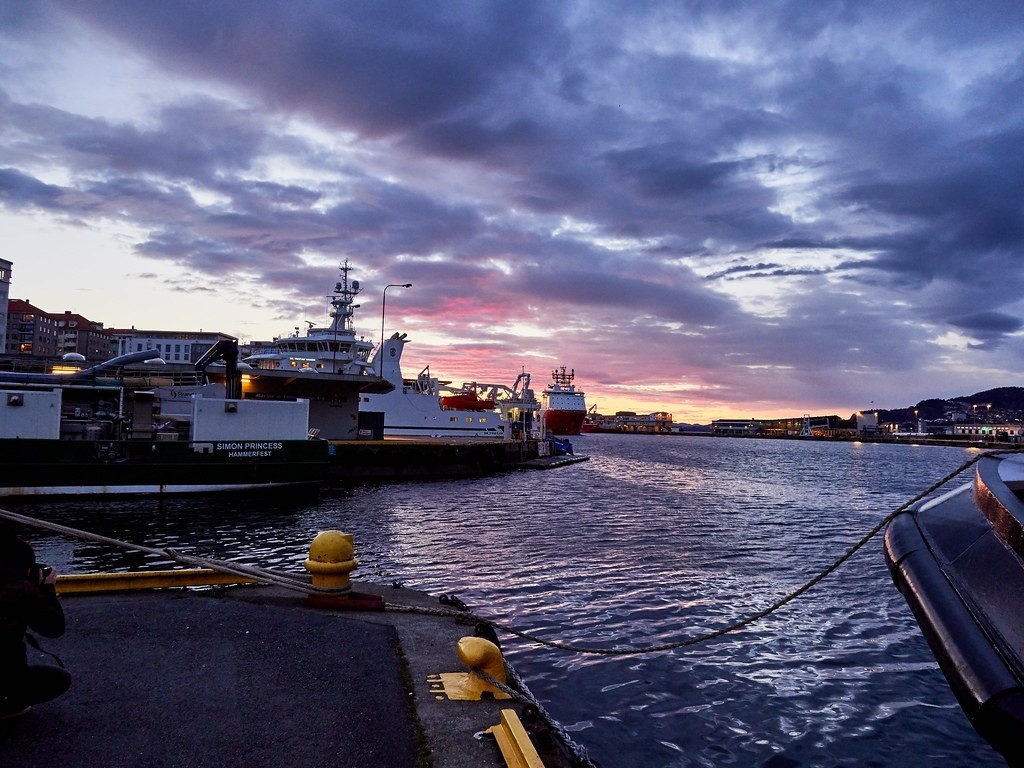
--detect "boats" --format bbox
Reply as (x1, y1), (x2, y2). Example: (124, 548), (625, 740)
(883, 447), (1023, 768)
(538, 365), (587, 436)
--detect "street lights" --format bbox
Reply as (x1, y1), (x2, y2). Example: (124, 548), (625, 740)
(332, 304), (360, 373)
(380, 283), (414, 375)
